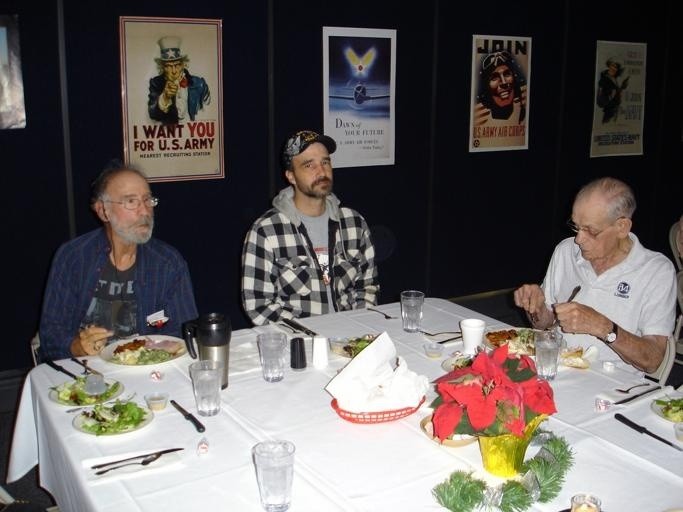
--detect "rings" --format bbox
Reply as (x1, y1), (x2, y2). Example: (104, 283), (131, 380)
(93, 345), (101, 352)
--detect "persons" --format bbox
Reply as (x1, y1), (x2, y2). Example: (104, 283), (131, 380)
(514, 177), (679, 385)
(238, 130), (382, 325)
(39, 165), (201, 368)
(595, 56), (631, 126)
(146, 34), (212, 125)
(474, 50), (528, 148)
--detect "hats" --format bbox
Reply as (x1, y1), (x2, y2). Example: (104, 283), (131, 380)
(154, 34), (188, 62)
(282, 129), (337, 160)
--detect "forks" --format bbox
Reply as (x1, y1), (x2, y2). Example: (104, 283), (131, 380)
(420, 328), (465, 338)
(367, 306), (398, 320)
(81, 323), (139, 340)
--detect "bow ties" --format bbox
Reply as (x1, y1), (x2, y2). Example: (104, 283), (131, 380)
(174, 78), (189, 90)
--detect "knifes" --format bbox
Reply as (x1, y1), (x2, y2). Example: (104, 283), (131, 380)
(71, 358), (100, 374)
(277, 314), (316, 337)
(436, 336), (464, 343)
(45, 360), (77, 379)
(614, 385), (662, 405)
(170, 399), (205, 433)
(615, 413), (683, 452)
(91, 448), (184, 470)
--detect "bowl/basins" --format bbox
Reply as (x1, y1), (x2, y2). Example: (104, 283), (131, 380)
(83, 374), (107, 395)
(673, 423), (683, 442)
(144, 391), (169, 411)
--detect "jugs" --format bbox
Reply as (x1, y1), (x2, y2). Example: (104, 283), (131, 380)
(187, 304), (232, 390)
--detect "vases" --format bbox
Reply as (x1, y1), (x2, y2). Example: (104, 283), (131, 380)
(478, 414), (547, 477)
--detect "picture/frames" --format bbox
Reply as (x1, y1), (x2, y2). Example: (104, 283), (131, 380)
(0, 13), (26, 130)
(120, 16), (224, 184)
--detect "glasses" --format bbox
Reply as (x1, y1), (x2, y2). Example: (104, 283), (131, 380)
(102, 196), (159, 210)
(566, 215), (628, 238)
(479, 49), (521, 75)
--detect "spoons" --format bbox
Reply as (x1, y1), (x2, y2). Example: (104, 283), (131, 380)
(615, 383), (650, 393)
(95, 451), (162, 476)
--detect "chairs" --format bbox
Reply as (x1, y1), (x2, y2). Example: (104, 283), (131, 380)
(30, 330), (41, 368)
(669, 223), (683, 270)
(644, 334), (676, 385)
(673, 271), (683, 365)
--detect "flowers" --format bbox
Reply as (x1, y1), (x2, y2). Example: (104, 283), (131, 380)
(429, 342), (557, 445)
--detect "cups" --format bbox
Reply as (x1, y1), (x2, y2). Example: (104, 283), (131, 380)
(399, 290), (424, 331)
(254, 329), (288, 386)
(459, 320), (485, 358)
(530, 331), (561, 381)
(251, 439), (295, 512)
(568, 491), (601, 512)
(187, 360), (225, 418)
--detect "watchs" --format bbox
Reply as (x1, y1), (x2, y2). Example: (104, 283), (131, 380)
(603, 321), (618, 344)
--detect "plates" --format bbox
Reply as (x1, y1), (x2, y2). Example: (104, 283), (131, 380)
(48, 377), (126, 407)
(485, 326), (569, 357)
(99, 334), (187, 366)
(648, 391), (683, 424)
(72, 403), (155, 436)
